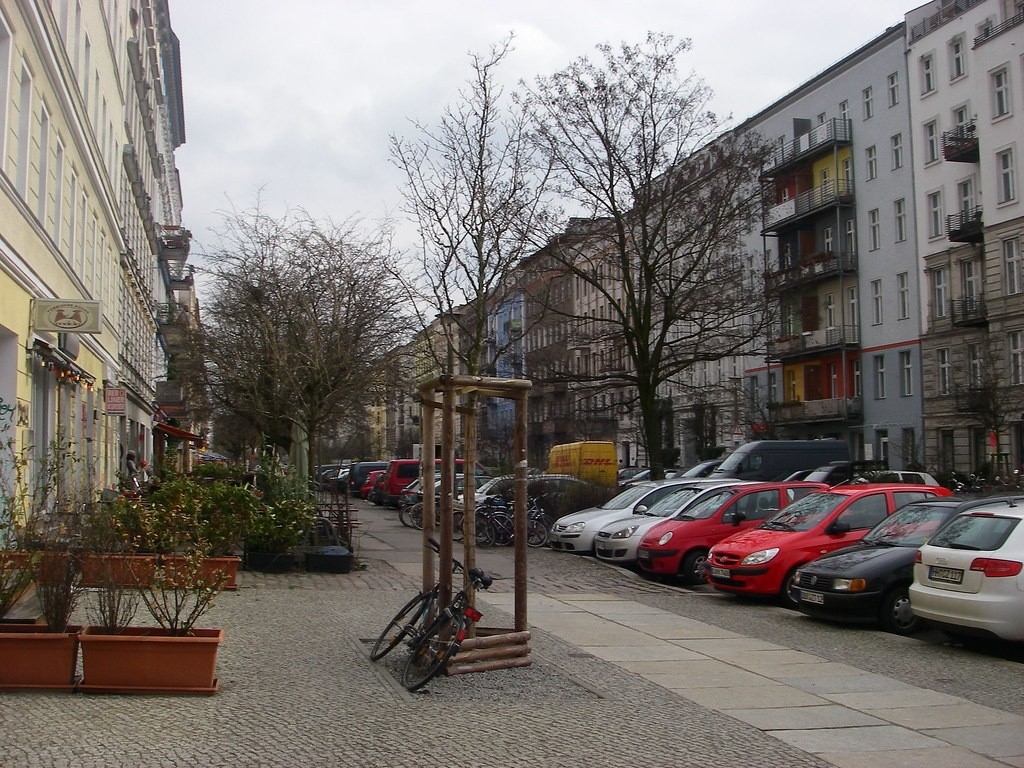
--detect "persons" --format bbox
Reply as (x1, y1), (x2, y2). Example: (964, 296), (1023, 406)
(126, 450), (141, 492)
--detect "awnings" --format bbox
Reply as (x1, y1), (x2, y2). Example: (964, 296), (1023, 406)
(153, 421), (204, 451)
(201, 451), (227, 463)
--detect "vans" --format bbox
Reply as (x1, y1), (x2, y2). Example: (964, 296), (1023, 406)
(382, 458), (497, 510)
(350, 462), (389, 492)
(705, 438), (849, 496)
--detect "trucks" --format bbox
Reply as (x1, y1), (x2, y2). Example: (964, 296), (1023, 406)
(543, 441), (618, 490)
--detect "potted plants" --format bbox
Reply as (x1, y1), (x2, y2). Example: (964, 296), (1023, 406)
(0, 496), (83, 694)
(0, 427), (80, 587)
(77, 500), (160, 587)
(77, 496), (231, 696)
(161, 477), (260, 590)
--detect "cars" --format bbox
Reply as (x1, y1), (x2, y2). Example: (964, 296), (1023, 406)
(786, 459), (940, 489)
(637, 480), (853, 586)
(594, 480), (772, 564)
(789, 496), (1024, 635)
(703, 483), (958, 610)
(368, 473), (385, 505)
(908, 500), (1024, 643)
(415, 475), (497, 509)
(400, 471), (468, 507)
(362, 470), (386, 497)
(618, 458), (724, 486)
(314, 464), (349, 492)
(457, 474), (602, 514)
(545, 476), (744, 552)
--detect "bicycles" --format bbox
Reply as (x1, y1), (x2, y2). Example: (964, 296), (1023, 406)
(370, 537), (493, 690)
(398, 490), (555, 548)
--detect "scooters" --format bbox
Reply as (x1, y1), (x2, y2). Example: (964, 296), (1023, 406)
(949, 468), (1024, 492)
